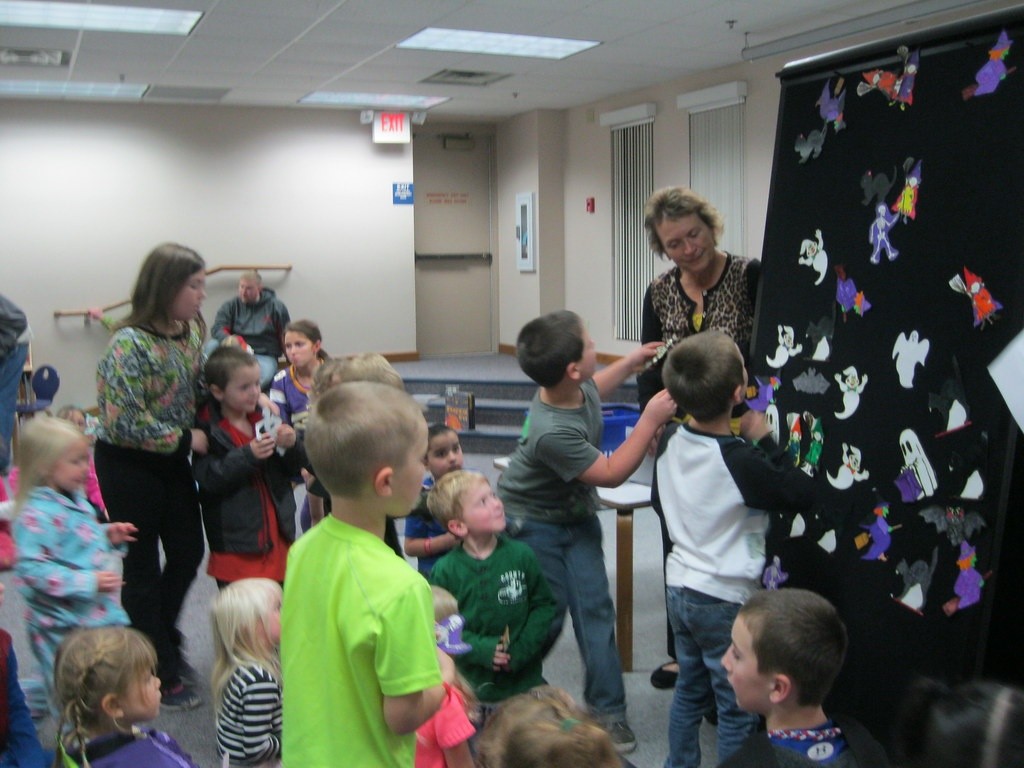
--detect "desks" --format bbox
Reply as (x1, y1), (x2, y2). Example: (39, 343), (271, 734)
(496, 423), (653, 671)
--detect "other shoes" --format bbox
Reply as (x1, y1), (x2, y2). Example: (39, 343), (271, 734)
(650, 661), (679, 688)
(605, 720), (636, 752)
(159, 678), (201, 711)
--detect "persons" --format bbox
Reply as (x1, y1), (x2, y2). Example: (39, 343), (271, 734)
(192, 347), (312, 593)
(636, 184), (760, 691)
(8, 404), (110, 518)
(306, 351), (405, 557)
(496, 310), (679, 752)
(270, 318), (333, 531)
(651, 330), (893, 768)
(98, 241), (281, 710)
(0, 415), (198, 767)
(878, 678), (1024, 768)
(210, 377), (625, 767)
(0, 292), (32, 477)
(211, 268), (292, 398)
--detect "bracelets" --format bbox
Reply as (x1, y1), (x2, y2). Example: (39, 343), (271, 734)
(423, 537), (434, 558)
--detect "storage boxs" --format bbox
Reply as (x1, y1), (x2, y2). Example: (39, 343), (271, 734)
(527, 402), (641, 459)
